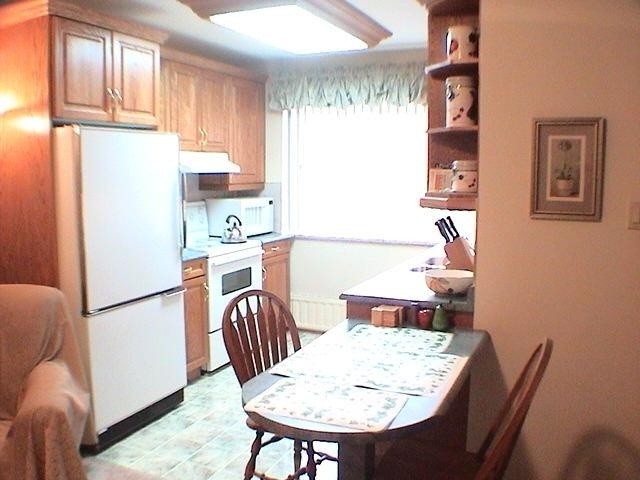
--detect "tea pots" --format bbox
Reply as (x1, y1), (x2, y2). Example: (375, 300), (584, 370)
(221, 215), (247, 244)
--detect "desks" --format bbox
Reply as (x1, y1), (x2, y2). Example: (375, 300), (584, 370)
(339, 244), (473, 330)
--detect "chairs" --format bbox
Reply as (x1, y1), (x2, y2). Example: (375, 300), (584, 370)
(373, 337), (555, 480)
(222, 290), (339, 480)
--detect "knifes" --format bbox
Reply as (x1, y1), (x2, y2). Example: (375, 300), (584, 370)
(441, 218), (456, 241)
(446, 216), (460, 237)
(438, 220), (450, 242)
(435, 222), (449, 243)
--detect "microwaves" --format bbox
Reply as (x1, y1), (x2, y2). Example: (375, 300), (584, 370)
(202, 197), (274, 238)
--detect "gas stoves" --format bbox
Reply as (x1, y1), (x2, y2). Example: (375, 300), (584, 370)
(184, 237), (262, 258)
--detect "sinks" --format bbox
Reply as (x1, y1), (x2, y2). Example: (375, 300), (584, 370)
(425, 256), (444, 266)
(411, 266), (439, 272)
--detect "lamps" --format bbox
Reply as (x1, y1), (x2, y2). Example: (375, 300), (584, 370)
(176, 0), (394, 56)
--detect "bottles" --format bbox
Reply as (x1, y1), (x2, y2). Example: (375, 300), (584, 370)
(410, 302), (420, 326)
(418, 309), (434, 330)
(432, 304), (449, 331)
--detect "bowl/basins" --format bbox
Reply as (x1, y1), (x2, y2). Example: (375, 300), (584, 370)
(425, 269), (474, 297)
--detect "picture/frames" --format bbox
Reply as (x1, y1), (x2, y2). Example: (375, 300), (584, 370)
(530, 116), (606, 222)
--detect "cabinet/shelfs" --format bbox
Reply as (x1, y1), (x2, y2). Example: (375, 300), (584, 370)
(0, 1), (161, 285)
(164, 49), (232, 152)
(198, 72), (267, 193)
(180, 252), (211, 384)
(262, 234), (296, 337)
(421, 0), (480, 209)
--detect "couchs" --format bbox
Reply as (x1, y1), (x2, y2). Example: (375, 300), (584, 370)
(0, 283), (89, 479)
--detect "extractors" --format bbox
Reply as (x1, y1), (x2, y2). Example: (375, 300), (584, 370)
(178, 150), (241, 175)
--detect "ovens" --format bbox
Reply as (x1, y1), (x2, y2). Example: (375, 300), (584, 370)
(201, 246), (262, 372)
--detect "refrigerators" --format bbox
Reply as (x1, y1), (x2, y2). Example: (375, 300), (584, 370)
(51, 123), (188, 456)
